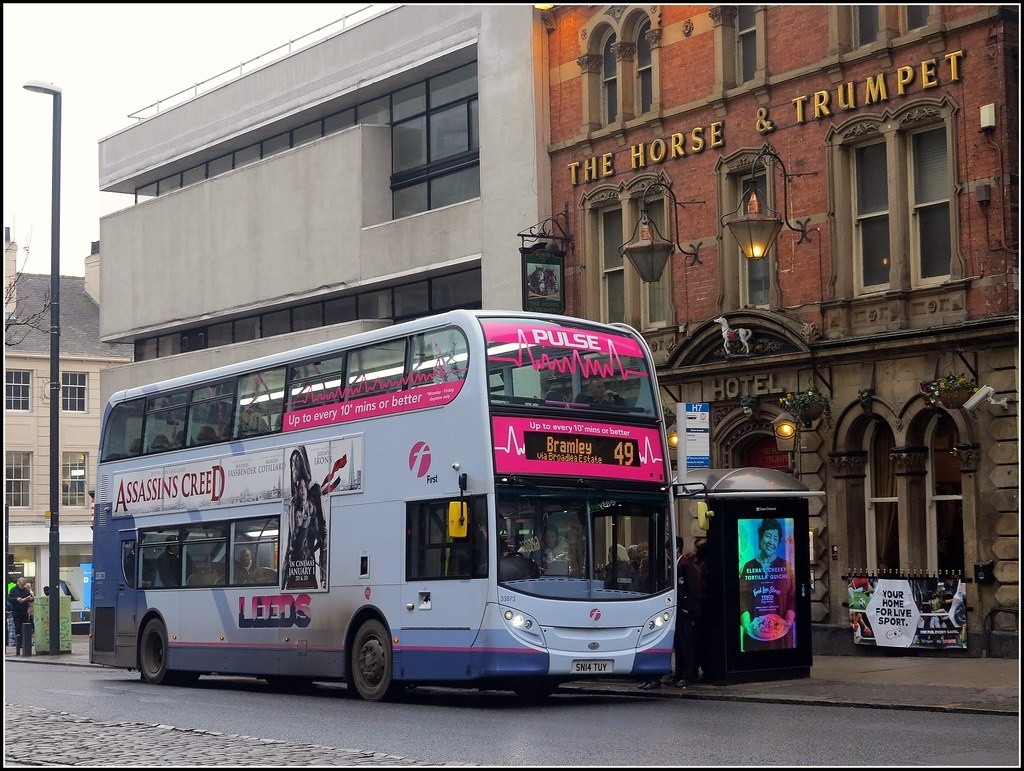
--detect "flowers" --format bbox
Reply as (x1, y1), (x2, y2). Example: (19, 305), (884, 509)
(780, 391), (834, 439)
(927, 373), (980, 403)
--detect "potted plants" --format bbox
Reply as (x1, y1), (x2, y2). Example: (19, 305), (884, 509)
(662, 406), (676, 428)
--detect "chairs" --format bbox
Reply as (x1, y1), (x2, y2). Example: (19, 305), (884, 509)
(142, 559), (158, 588)
(185, 564), (277, 585)
(544, 390), (584, 403)
(129, 412), (284, 455)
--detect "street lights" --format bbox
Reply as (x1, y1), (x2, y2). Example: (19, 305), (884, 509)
(23, 80), (63, 657)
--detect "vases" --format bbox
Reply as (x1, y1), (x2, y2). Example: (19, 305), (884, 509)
(939, 390), (970, 409)
(798, 404), (824, 421)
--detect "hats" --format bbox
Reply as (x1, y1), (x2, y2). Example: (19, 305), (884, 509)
(695, 539), (706, 546)
(609, 544), (630, 561)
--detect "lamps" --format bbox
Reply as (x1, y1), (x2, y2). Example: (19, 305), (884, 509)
(721, 144), (818, 261)
(618, 175), (707, 284)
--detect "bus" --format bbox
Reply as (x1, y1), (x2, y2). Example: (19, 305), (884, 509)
(86, 309), (715, 705)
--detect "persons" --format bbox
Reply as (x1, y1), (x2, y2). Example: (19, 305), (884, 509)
(236, 547), (260, 587)
(848, 577), (880, 644)
(601, 535), (712, 691)
(738, 517), (798, 651)
(472, 513), (514, 570)
(284, 445), (329, 591)
(8, 578), (51, 655)
(540, 526), (573, 574)
(142, 536), (197, 589)
